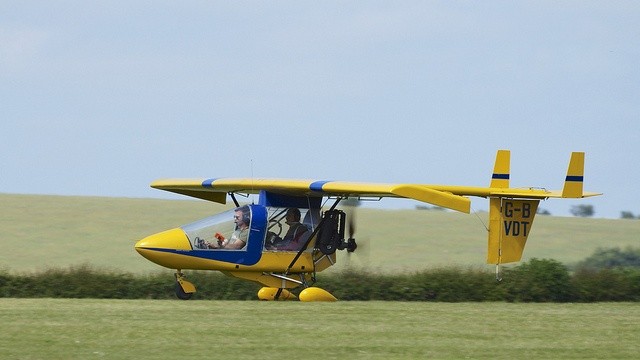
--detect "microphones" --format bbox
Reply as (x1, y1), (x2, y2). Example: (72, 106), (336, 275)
(284, 221), (298, 223)
(293, 209), (300, 220)
(234, 219), (242, 223)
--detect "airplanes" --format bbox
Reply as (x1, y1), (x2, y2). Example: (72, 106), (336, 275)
(132, 151), (603, 302)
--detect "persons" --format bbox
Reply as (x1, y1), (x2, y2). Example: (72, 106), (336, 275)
(266, 208), (310, 250)
(201, 206), (250, 250)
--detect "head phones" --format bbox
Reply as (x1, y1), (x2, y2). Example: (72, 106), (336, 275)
(242, 206), (251, 223)
